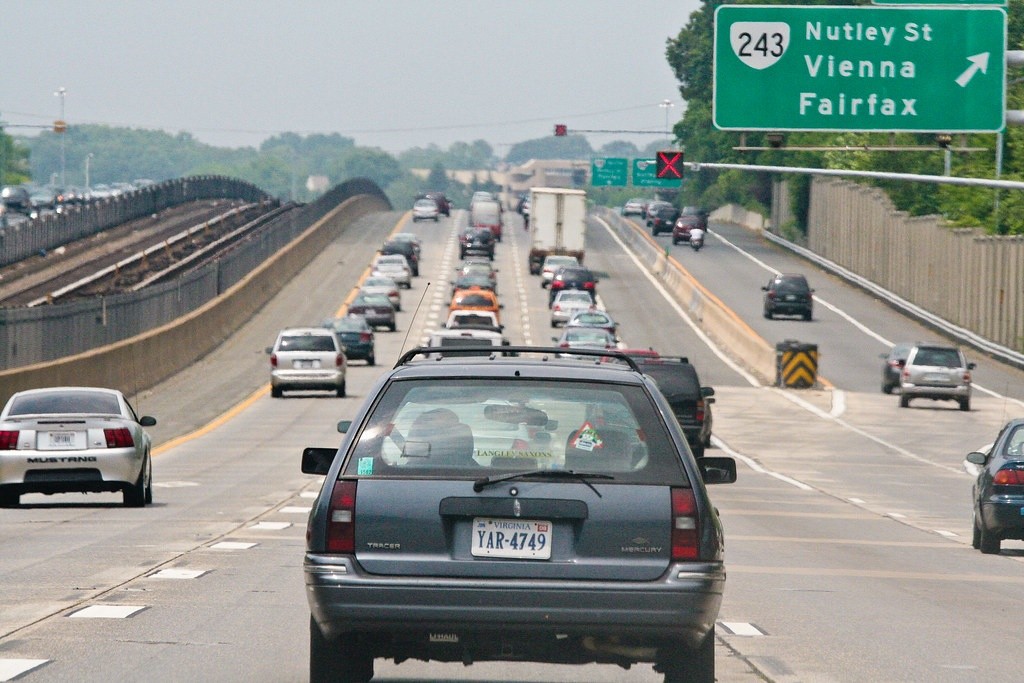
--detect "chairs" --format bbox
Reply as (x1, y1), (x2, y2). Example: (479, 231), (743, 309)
(405, 419), (479, 465)
(561, 428), (634, 471)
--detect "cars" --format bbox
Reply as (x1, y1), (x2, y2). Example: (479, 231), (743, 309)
(296, 346), (736, 683)
(962, 418), (1024, 557)
(877, 342), (911, 394)
(897, 341), (977, 410)
(760, 273), (815, 322)
(0, 387), (155, 507)
(0, 177), (159, 226)
(265, 188), (711, 456)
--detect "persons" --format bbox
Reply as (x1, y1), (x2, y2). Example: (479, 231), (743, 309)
(406, 409), (479, 467)
(689, 225), (704, 248)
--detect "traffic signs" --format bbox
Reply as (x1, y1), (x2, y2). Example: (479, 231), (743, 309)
(712, 5), (1006, 134)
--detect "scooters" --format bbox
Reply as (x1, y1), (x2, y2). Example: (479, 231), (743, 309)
(691, 240), (702, 251)
